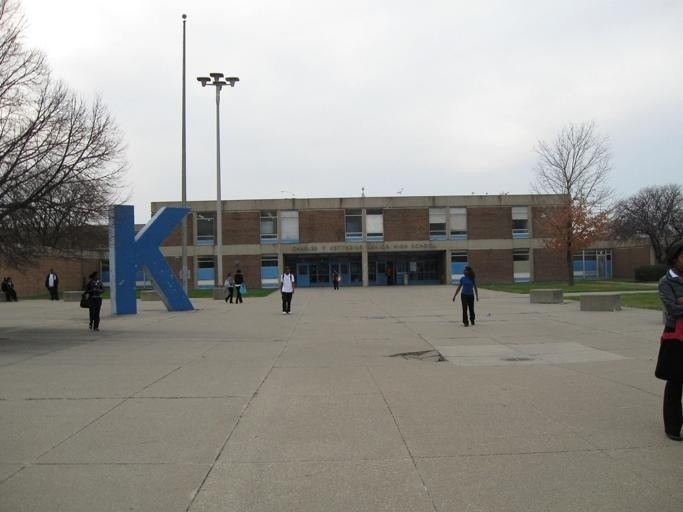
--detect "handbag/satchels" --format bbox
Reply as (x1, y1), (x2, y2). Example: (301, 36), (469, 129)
(80, 292), (90, 308)
(240, 286), (246, 294)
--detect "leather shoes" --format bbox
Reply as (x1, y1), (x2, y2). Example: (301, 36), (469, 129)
(666, 433), (683, 441)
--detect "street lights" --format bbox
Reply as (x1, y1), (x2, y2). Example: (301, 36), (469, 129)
(197, 72), (242, 299)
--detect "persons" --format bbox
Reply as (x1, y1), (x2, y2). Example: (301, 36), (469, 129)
(5, 275), (18, 303)
(224, 272), (234, 304)
(279, 266), (295, 316)
(332, 268), (339, 290)
(452, 266), (479, 328)
(83, 271), (106, 332)
(45, 267), (60, 301)
(657, 243), (682, 442)
(0, 277), (6, 292)
(234, 269), (245, 305)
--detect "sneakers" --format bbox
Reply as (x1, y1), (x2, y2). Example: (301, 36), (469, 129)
(464, 324), (468, 327)
(283, 311), (291, 315)
(94, 328), (98, 331)
(230, 302), (233, 304)
(471, 320), (474, 325)
(89, 324), (92, 329)
(224, 297), (227, 302)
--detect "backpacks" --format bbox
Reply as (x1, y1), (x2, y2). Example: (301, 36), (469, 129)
(224, 279), (230, 289)
